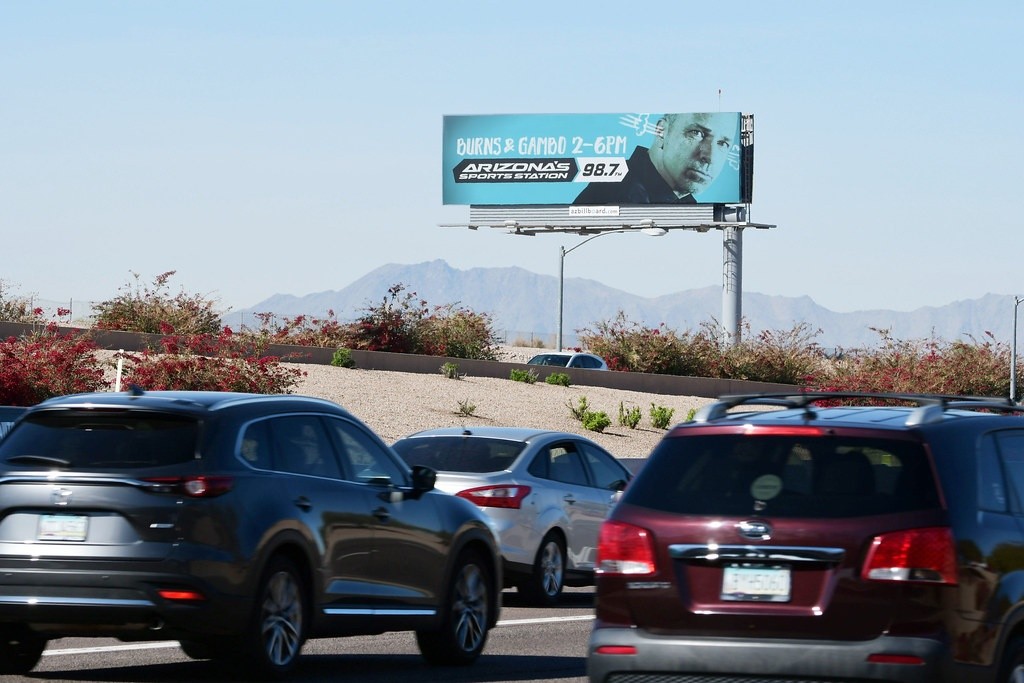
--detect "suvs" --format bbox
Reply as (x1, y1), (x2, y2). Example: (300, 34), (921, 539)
(584, 392), (1024, 682)
(0, 393), (504, 683)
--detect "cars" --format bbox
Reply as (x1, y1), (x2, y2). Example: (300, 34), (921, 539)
(357, 425), (616, 594)
(526, 352), (608, 371)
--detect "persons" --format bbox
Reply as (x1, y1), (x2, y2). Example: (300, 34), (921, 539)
(572, 112), (737, 206)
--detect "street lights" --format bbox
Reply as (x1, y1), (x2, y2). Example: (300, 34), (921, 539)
(557, 227), (671, 351)
